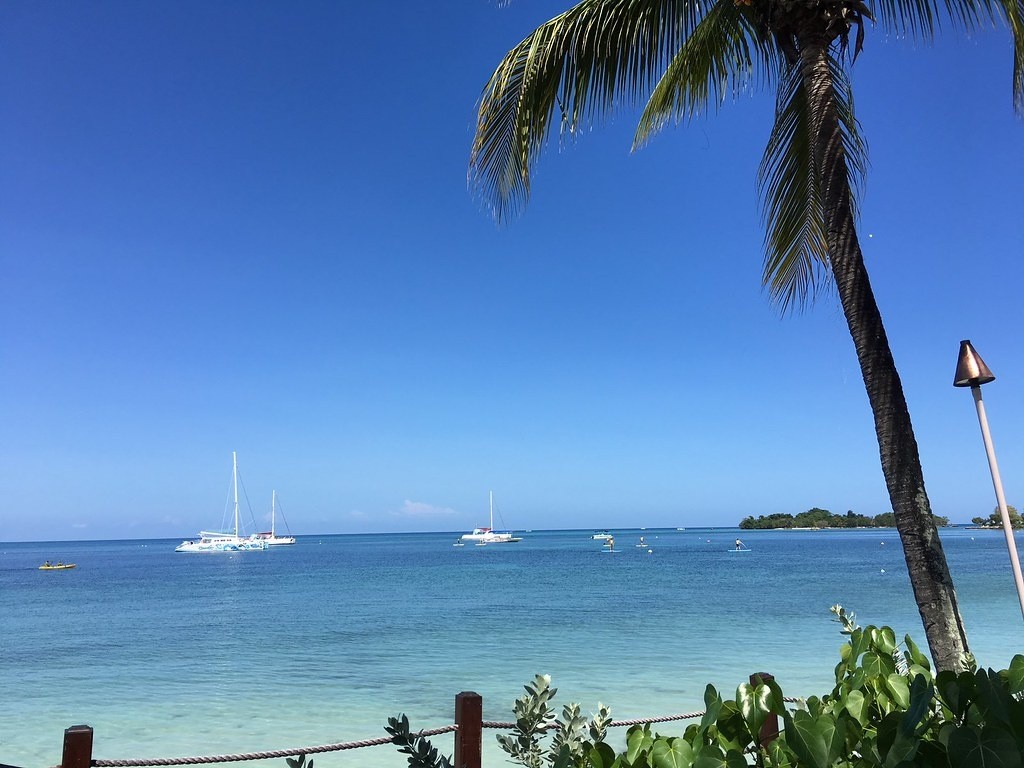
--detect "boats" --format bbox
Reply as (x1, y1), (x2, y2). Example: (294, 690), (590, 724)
(39, 564), (76, 570)
(591, 534), (613, 540)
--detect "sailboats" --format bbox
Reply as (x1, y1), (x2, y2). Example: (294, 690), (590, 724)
(461, 490), (512, 541)
(249, 488), (296, 546)
(174, 451), (269, 553)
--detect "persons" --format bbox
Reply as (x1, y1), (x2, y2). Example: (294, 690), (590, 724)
(44, 559), (51, 567)
(608, 537), (615, 551)
(736, 538), (740, 550)
(640, 535), (644, 545)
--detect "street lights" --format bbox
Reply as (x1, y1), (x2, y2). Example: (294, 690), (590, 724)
(951, 338), (1024, 620)
